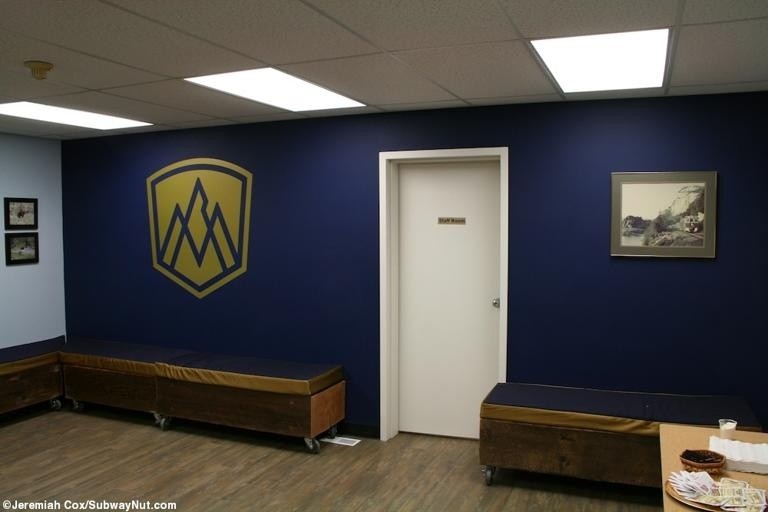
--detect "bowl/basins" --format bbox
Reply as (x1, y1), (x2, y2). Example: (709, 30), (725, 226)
(679, 449), (727, 474)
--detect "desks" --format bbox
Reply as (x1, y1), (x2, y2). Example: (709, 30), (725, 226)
(658, 422), (767, 511)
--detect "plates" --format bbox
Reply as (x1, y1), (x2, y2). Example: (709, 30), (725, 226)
(664, 475), (768, 512)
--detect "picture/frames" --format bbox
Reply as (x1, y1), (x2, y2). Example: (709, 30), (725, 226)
(4, 197), (38, 230)
(5, 233), (39, 265)
(608, 172), (716, 258)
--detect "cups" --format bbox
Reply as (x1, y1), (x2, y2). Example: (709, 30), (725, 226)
(719, 418), (737, 440)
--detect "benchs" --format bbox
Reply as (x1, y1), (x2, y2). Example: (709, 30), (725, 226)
(59, 346), (346, 454)
(1, 347), (65, 411)
(479, 383), (767, 488)
